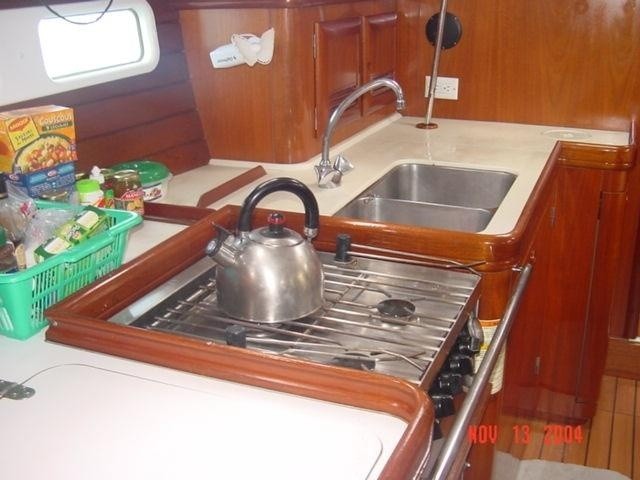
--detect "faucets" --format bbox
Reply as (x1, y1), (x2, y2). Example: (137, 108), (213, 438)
(314, 76), (406, 183)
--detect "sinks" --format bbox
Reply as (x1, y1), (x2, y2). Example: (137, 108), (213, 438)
(362, 161), (517, 210)
(329, 194), (495, 235)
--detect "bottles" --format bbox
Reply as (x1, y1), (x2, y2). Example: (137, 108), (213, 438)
(0, 225), (19, 275)
(76, 180), (104, 206)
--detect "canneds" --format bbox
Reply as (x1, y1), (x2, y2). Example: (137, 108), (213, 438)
(39, 190), (68, 202)
(113, 169), (145, 216)
(98, 169), (116, 209)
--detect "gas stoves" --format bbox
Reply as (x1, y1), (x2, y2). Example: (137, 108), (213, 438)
(106, 247), (483, 441)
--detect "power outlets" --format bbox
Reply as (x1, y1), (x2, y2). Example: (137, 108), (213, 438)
(424, 76), (459, 101)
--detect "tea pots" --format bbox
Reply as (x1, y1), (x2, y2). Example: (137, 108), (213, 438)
(207, 177), (326, 323)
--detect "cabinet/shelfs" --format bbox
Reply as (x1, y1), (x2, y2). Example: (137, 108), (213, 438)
(179, 2), (399, 167)
